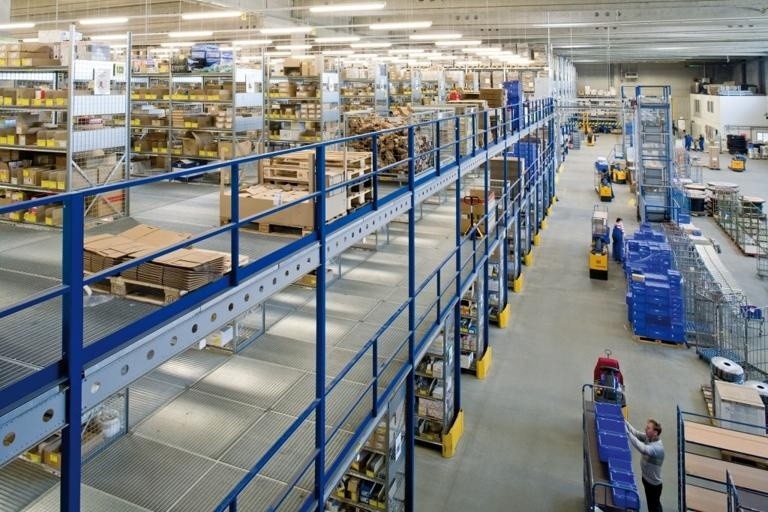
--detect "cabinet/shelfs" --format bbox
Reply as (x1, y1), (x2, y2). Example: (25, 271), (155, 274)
(675, 407), (768, 511)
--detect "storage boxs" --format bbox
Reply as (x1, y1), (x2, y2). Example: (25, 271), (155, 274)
(595, 403), (640, 510)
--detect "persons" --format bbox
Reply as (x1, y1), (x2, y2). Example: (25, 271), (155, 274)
(611, 217), (624, 261)
(698, 134), (704, 151)
(624, 416), (664, 512)
(684, 134), (693, 150)
